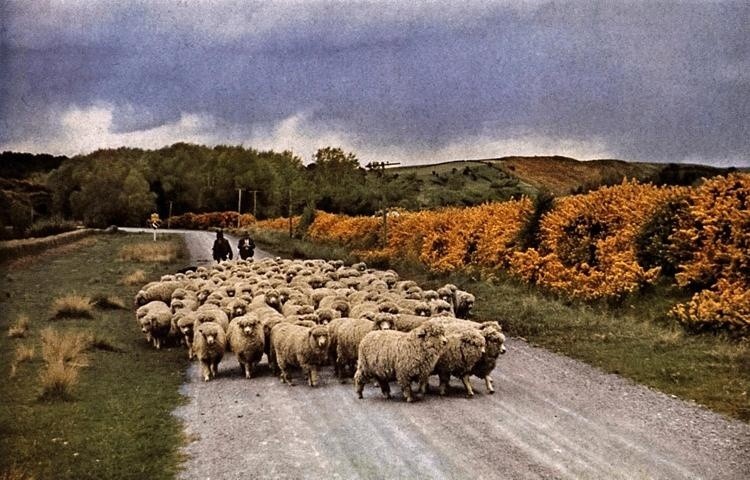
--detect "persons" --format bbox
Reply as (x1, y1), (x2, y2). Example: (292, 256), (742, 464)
(237, 230), (256, 260)
(211, 228), (234, 264)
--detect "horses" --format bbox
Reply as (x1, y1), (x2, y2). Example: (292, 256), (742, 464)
(215, 229), (227, 263)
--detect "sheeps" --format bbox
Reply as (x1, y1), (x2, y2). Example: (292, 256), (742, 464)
(133, 255), (506, 403)
